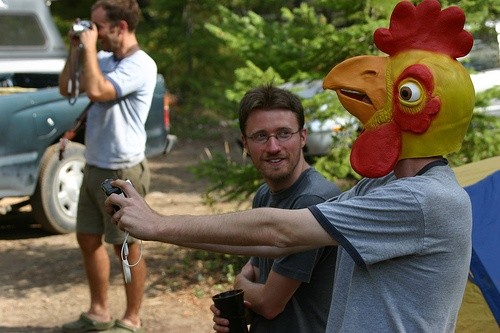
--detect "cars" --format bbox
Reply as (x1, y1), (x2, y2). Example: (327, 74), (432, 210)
(0, 0), (66, 59)
(272, 22), (500, 156)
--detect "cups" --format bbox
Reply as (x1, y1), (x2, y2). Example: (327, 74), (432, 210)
(212, 289), (249, 333)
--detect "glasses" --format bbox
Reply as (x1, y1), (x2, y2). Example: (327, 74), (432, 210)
(245, 129), (299, 141)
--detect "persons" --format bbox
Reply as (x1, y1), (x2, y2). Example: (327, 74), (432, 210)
(64, 0), (158, 333)
(105, 0), (474, 333)
(210, 86), (340, 333)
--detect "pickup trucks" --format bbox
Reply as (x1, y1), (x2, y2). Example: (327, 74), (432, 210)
(0, 53), (176, 233)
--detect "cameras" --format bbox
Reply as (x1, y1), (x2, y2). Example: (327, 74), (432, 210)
(72, 21), (91, 37)
(101, 179), (133, 212)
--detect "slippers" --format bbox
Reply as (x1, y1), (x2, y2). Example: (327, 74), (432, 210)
(96, 319), (145, 333)
(61, 312), (114, 333)
